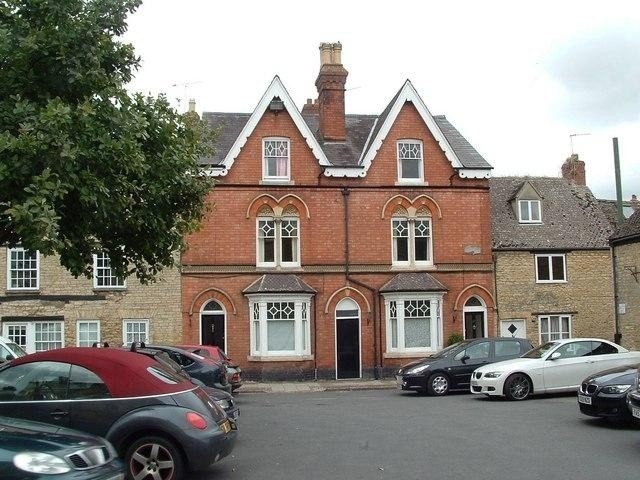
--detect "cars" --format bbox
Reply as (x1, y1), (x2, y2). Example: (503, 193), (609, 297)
(393, 337), (640, 420)
(0, 334), (243, 479)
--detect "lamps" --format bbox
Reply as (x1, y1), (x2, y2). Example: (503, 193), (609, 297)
(270, 101), (284, 115)
